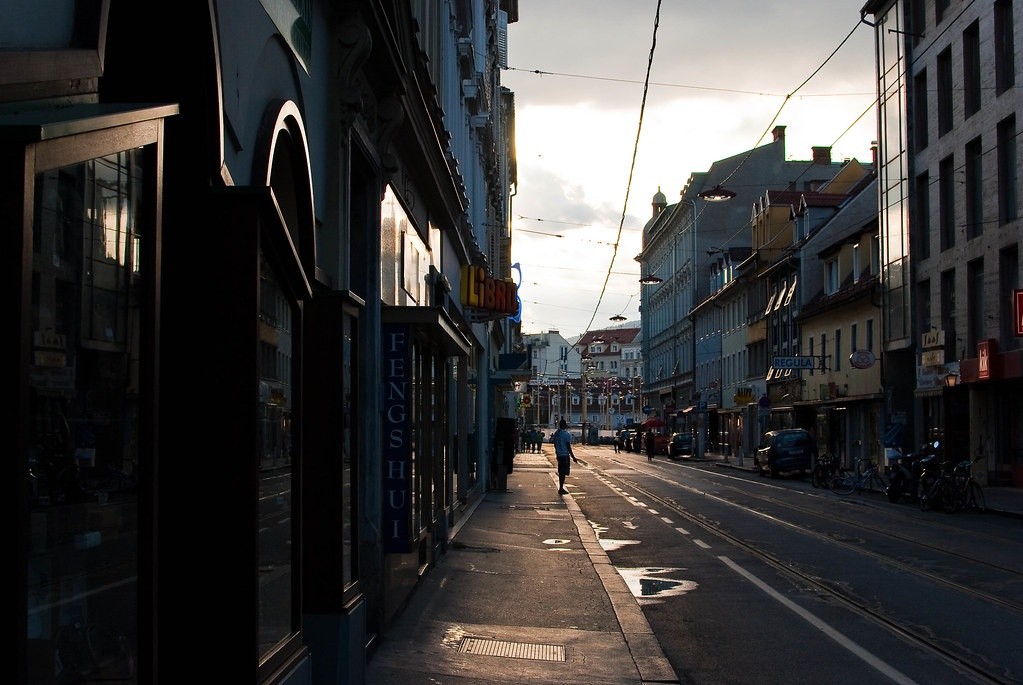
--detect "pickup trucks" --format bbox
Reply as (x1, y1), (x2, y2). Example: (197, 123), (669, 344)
(640, 433), (673, 455)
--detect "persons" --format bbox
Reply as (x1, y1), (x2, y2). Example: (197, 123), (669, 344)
(613, 427), (644, 454)
(554, 420), (580, 494)
(646, 428), (655, 462)
(549, 433), (554, 443)
(519, 429), (543, 453)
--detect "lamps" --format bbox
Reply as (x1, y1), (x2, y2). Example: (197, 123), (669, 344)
(944, 369), (958, 390)
(697, 186), (737, 201)
(609, 315), (627, 322)
(638, 273), (664, 285)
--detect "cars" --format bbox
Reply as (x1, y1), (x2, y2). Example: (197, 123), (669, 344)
(624, 433), (637, 450)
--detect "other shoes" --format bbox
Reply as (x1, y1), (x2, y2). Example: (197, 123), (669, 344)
(558, 490), (569, 494)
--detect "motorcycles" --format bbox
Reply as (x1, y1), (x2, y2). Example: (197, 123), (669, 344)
(888, 442), (940, 499)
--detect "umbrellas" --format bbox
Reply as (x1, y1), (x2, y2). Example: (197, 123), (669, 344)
(641, 418), (666, 435)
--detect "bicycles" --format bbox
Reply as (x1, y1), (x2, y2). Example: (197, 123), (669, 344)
(811, 450), (888, 496)
(931, 455), (986, 513)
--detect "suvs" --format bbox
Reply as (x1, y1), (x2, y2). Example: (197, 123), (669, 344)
(666, 432), (697, 459)
(757, 428), (819, 479)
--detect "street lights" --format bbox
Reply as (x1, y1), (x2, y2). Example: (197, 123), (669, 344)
(534, 361), (643, 431)
